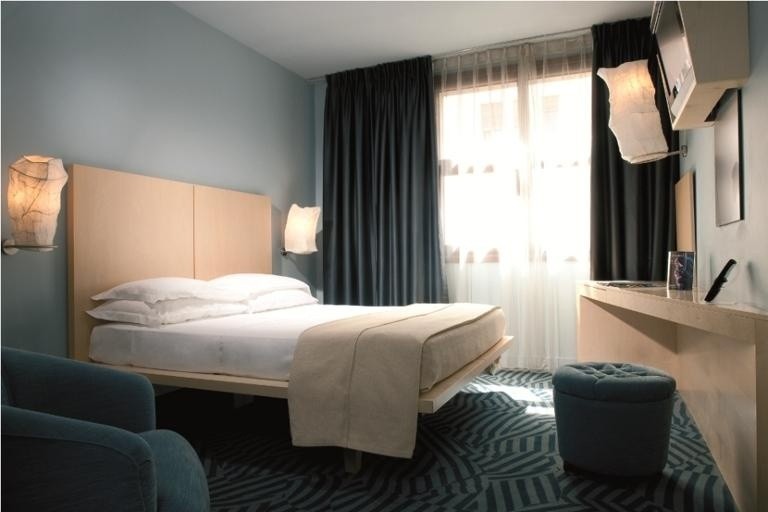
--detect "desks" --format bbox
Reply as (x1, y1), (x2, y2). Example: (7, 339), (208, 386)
(574, 274), (768, 510)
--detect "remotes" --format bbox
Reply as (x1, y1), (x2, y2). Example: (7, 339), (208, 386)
(704, 258), (736, 302)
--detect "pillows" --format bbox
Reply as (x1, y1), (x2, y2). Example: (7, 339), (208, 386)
(84, 271), (320, 328)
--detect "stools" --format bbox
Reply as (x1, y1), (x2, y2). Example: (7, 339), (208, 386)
(550, 358), (678, 480)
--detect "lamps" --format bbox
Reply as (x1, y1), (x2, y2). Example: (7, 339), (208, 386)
(2, 154), (70, 256)
(596, 59), (687, 165)
(280, 200), (322, 258)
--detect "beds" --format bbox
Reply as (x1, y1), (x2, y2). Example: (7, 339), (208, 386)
(65, 157), (517, 476)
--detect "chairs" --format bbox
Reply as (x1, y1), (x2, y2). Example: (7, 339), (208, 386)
(1, 346), (212, 510)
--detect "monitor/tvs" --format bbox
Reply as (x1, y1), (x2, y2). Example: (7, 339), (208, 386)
(650, 1), (751, 131)
